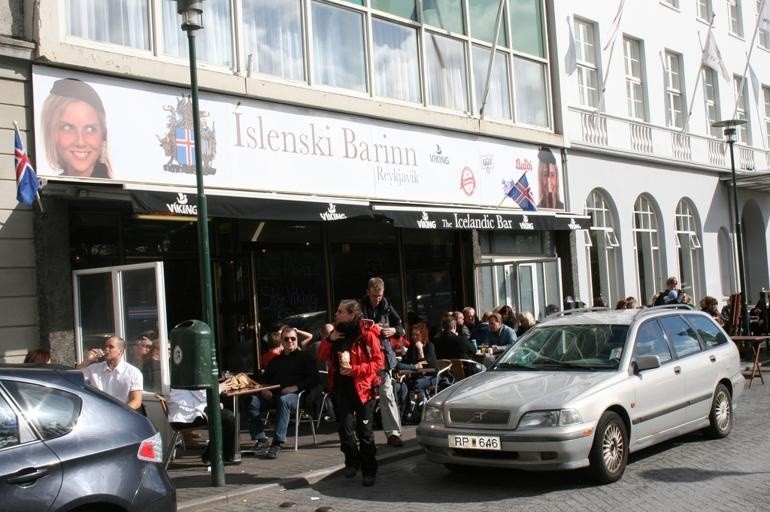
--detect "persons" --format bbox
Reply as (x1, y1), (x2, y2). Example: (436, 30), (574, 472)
(536, 147), (563, 209)
(358, 277), (405, 447)
(244, 321), (334, 460)
(77, 336), (144, 409)
(167, 379), (240, 463)
(392, 305), (560, 402)
(41, 78), (113, 179)
(141, 338), (207, 438)
(129, 338), (153, 370)
(699, 294), (770, 337)
(651, 277), (693, 309)
(614, 296), (639, 310)
(23, 348), (52, 365)
(318, 300), (385, 488)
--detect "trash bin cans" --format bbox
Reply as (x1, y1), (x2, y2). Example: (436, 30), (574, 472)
(169, 320), (214, 391)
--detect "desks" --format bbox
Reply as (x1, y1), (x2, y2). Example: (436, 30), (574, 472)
(730, 335), (770, 389)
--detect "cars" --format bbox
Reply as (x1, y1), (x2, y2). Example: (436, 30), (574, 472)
(85, 331), (115, 345)
(0, 358), (177, 512)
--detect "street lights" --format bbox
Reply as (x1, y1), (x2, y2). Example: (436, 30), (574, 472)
(713, 118), (754, 355)
(175, 0), (228, 491)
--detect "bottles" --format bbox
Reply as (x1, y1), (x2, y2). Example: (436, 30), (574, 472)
(471, 340), (477, 351)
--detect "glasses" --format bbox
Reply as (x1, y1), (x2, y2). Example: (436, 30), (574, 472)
(284, 336), (296, 341)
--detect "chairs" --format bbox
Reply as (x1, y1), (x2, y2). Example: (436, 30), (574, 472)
(137, 342), (502, 472)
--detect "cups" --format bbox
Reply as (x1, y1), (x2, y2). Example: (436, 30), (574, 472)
(221, 370), (229, 381)
(338, 352), (351, 367)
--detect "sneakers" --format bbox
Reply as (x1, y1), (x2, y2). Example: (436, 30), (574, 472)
(345, 465), (357, 477)
(251, 440), (271, 451)
(388, 436), (404, 446)
(266, 446), (280, 458)
(362, 472), (376, 486)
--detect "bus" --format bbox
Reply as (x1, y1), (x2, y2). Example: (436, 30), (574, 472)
(408, 292), (453, 311)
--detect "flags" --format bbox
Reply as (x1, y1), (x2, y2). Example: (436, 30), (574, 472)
(14, 131), (40, 208)
(175, 128), (197, 167)
(507, 174), (537, 211)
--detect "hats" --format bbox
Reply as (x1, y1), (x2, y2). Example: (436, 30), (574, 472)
(539, 148), (556, 163)
(50, 78), (105, 113)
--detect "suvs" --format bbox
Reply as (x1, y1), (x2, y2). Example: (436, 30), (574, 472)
(417, 302), (747, 485)
(265, 310), (332, 342)
